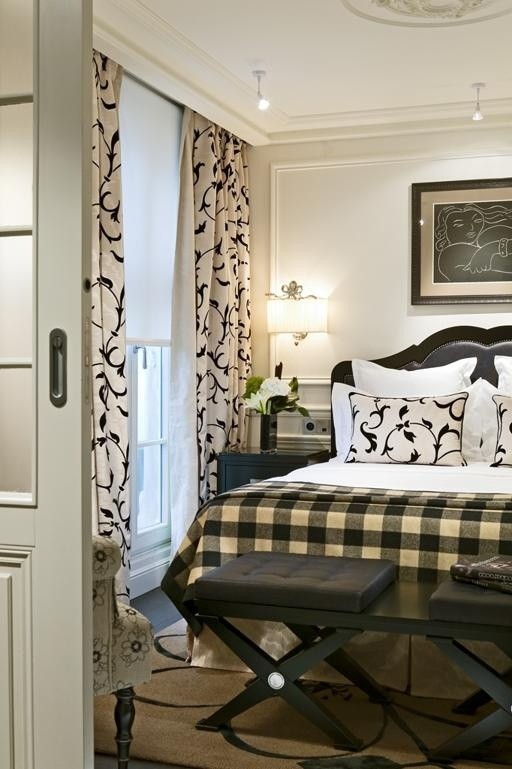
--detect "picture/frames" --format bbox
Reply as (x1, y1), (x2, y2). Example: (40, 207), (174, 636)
(403, 176), (511, 307)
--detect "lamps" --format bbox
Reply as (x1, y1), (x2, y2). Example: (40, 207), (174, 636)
(342, 0), (512, 35)
(469, 82), (485, 122)
(263, 279), (331, 345)
(249, 69), (271, 113)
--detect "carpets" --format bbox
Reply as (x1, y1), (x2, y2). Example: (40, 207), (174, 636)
(92, 613), (512, 766)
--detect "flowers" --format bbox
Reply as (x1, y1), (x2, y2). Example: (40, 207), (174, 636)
(234, 359), (311, 420)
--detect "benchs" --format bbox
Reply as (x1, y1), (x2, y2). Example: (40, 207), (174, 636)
(180, 571), (509, 762)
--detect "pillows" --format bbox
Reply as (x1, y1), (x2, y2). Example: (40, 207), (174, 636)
(491, 351), (511, 393)
(330, 375), (482, 461)
(347, 355), (477, 390)
(488, 394), (511, 467)
(482, 378), (511, 460)
(343, 389), (469, 470)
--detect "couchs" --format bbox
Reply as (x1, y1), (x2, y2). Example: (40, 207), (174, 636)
(89, 535), (160, 767)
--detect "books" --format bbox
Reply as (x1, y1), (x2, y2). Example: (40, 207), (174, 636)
(450, 555), (511, 583)
(450, 573), (511, 594)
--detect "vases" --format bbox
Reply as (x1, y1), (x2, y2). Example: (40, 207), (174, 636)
(258, 412), (278, 454)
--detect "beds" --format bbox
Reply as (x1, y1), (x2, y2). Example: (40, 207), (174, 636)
(188, 324), (512, 693)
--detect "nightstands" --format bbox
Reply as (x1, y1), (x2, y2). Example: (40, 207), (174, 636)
(215, 444), (333, 500)
